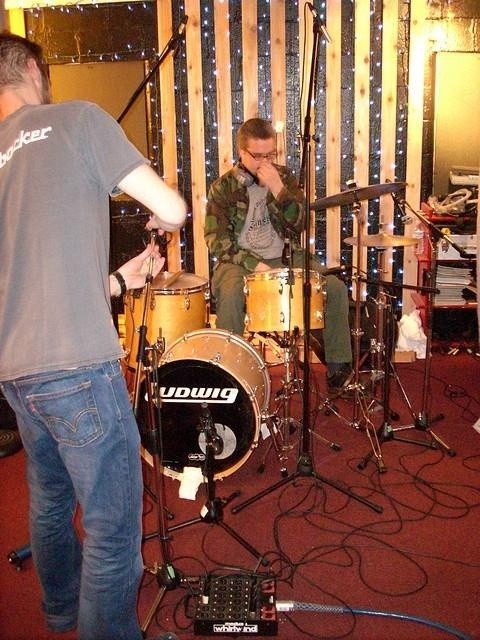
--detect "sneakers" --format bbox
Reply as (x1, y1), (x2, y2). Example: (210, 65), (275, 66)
(326, 365), (368, 400)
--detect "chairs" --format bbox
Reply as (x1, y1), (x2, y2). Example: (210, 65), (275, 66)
(207, 248), (319, 394)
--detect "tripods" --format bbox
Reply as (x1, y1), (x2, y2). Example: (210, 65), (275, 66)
(8, 23), (457, 640)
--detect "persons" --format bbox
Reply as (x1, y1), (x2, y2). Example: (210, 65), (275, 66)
(0, 29), (188, 640)
(204, 118), (354, 395)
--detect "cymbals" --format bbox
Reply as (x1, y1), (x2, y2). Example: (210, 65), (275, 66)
(345, 235), (419, 247)
(311, 182), (407, 209)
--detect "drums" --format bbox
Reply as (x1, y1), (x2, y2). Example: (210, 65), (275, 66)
(244, 268), (325, 333)
(121, 271), (207, 372)
(133, 329), (272, 483)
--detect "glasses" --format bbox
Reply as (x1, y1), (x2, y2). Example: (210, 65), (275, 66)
(243, 146), (276, 161)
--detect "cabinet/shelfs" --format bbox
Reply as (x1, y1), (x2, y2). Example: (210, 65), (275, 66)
(428, 214), (480, 353)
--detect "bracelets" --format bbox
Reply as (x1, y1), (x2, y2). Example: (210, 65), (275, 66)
(112, 271), (127, 297)
